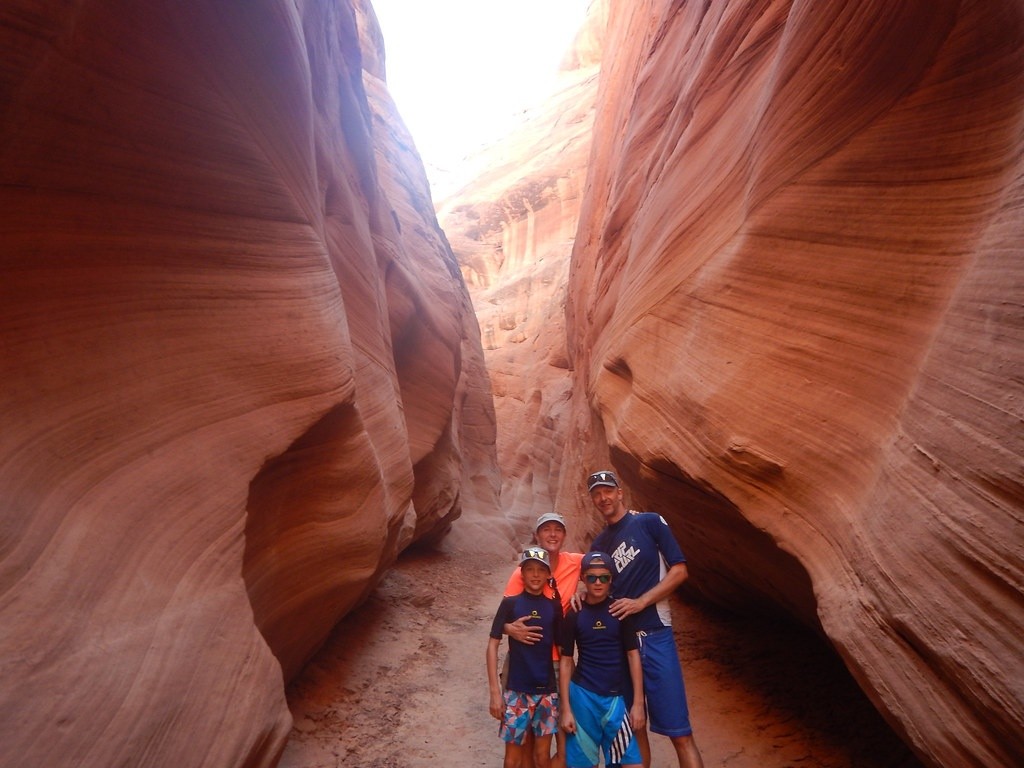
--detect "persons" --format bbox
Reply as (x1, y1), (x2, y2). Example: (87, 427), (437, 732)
(503, 513), (639, 768)
(559, 552), (645, 768)
(570, 471), (703, 768)
(486, 549), (575, 768)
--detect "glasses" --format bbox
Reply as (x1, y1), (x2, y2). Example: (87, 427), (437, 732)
(586, 473), (620, 489)
(583, 573), (614, 584)
(521, 564), (548, 574)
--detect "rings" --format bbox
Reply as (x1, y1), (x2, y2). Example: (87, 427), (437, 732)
(526, 636), (529, 640)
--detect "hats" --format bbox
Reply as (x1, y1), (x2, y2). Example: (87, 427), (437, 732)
(588, 471), (619, 491)
(535, 512), (566, 533)
(580, 551), (616, 579)
(519, 546), (550, 567)
